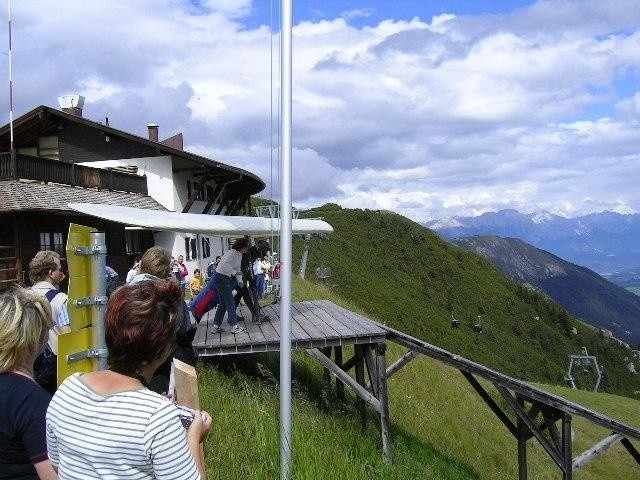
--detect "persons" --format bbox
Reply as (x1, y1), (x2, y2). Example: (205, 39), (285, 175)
(126, 236), (275, 336)
(46, 278), (215, 480)
(25, 249), (71, 399)
(0, 288), (60, 480)
(125, 245), (199, 399)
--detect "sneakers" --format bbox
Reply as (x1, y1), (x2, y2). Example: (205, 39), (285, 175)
(232, 325), (244, 334)
(210, 327), (225, 335)
(251, 313), (270, 326)
(226, 316), (244, 323)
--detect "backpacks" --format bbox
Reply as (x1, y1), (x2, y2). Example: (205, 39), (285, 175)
(32, 288), (61, 387)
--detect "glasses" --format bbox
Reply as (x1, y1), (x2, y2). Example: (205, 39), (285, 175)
(54, 268), (64, 274)
(42, 320), (58, 332)
